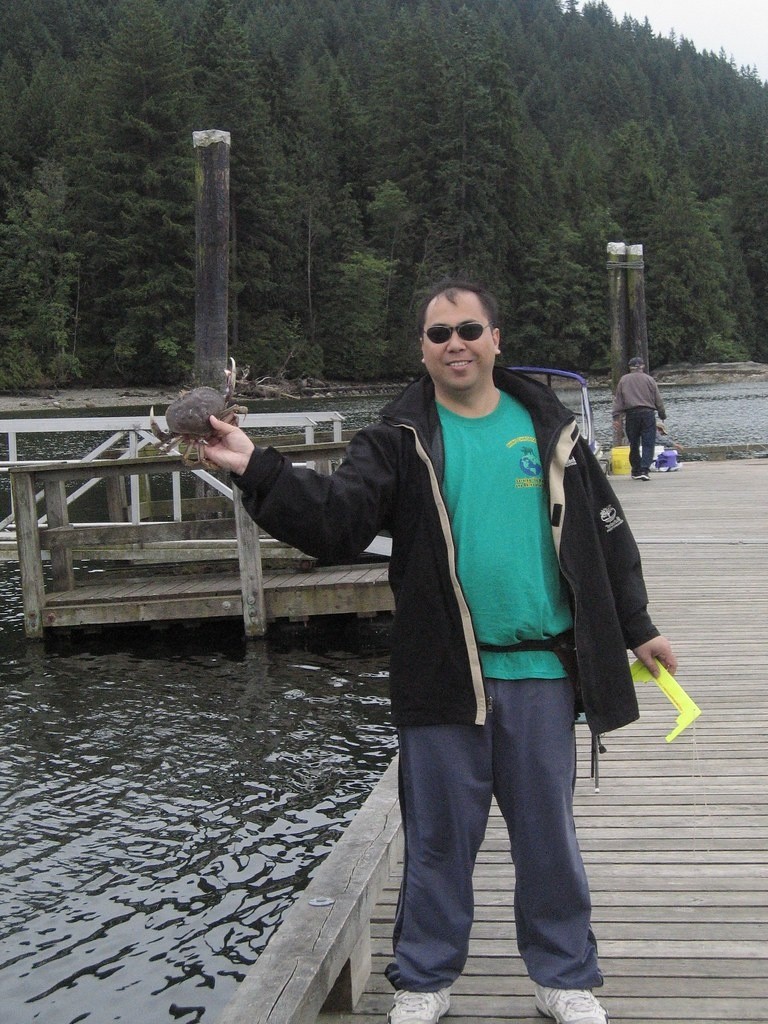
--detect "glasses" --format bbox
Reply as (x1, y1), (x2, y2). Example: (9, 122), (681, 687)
(424, 321), (493, 344)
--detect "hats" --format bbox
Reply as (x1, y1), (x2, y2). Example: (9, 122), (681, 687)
(628, 357), (645, 365)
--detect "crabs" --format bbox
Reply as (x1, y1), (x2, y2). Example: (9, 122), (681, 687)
(150, 357), (249, 469)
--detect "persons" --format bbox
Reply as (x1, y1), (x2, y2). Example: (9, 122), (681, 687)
(194, 281), (678, 1024)
(611, 357), (667, 480)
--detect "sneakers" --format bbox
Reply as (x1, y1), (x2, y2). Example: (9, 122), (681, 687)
(641, 471), (651, 480)
(631, 472), (641, 479)
(387, 986), (450, 1024)
(534, 983), (610, 1024)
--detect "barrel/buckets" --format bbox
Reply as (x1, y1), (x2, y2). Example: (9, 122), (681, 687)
(612, 446), (632, 474)
(662, 448), (677, 468)
